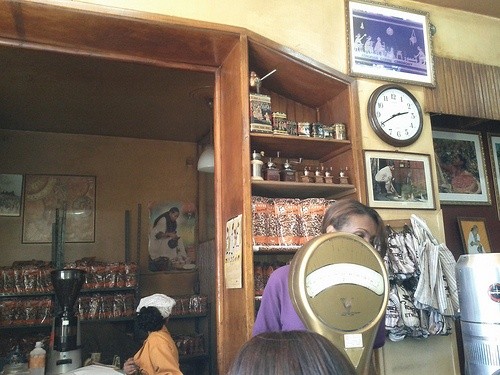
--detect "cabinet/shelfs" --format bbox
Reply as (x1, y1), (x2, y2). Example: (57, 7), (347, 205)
(214, 37), (382, 375)
(0, 202), (212, 375)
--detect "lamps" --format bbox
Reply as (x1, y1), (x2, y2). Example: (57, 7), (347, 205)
(196, 98), (215, 174)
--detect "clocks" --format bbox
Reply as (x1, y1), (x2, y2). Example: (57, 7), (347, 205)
(369, 83), (423, 148)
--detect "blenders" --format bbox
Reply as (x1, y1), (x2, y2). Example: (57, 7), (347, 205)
(45, 268), (86, 375)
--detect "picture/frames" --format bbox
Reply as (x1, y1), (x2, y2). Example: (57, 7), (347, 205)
(0, 172), (24, 217)
(431, 127), (493, 206)
(21, 173), (97, 245)
(487, 132), (500, 220)
(456, 215), (494, 253)
(363, 149), (436, 211)
(344, 0), (437, 90)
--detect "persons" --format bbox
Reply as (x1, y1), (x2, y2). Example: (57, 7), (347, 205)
(374, 162), (398, 195)
(468, 225), (486, 253)
(252, 198), (388, 375)
(148, 207), (191, 261)
(225, 330), (357, 375)
(123, 293), (185, 375)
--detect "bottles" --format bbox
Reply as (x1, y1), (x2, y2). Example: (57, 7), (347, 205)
(30, 341), (47, 375)
